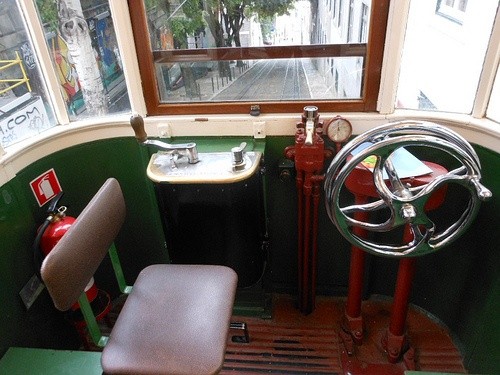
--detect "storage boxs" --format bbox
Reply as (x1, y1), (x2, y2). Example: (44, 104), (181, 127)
(0, 346), (104, 375)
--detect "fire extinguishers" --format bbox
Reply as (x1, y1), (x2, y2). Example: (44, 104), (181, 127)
(33, 191), (98, 311)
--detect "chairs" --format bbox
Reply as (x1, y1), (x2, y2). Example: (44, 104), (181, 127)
(39, 176), (239, 375)
